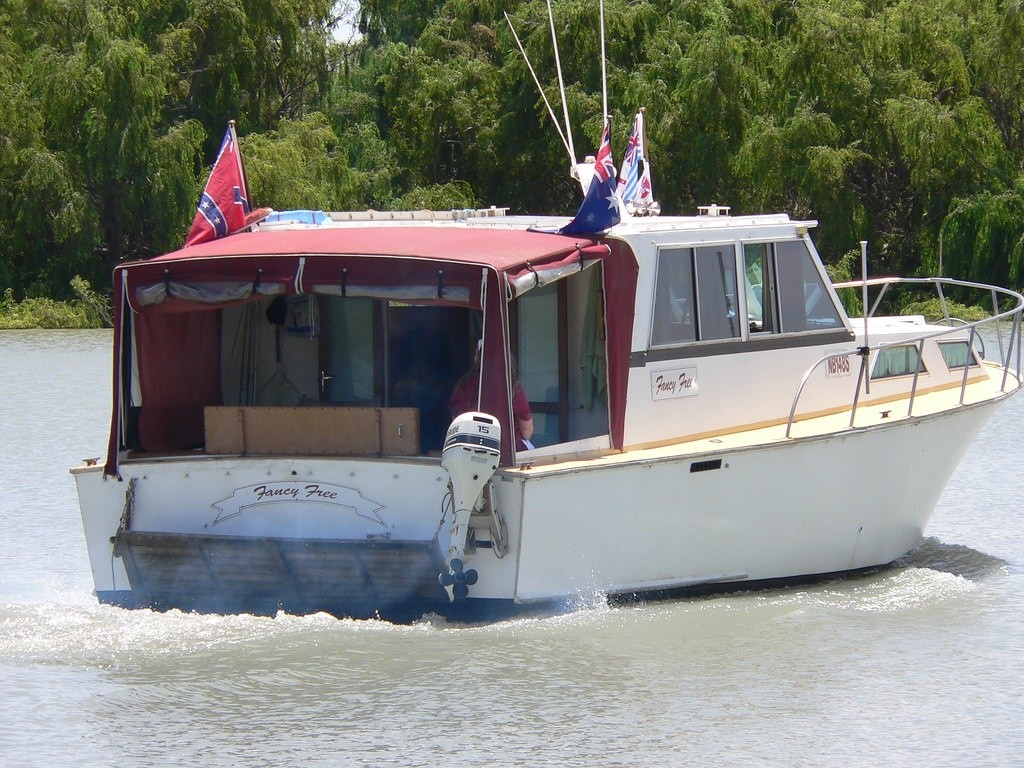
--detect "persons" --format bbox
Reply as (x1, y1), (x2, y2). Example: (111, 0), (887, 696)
(449, 345), (534, 470)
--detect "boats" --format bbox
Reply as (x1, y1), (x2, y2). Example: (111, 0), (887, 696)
(66, 1), (1024, 626)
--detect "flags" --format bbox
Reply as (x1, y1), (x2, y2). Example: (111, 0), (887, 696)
(615, 113), (643, 204)
(526, 123), (620, 235)
(184, 127), (249, 249)
(636, 160), (653, 202)
